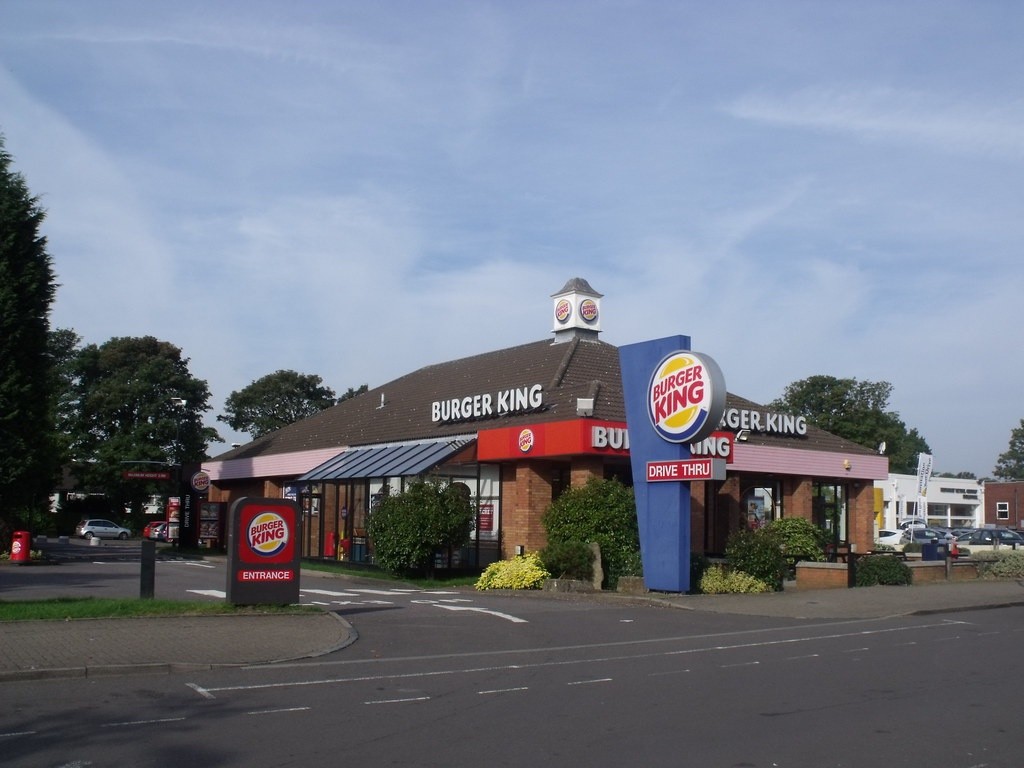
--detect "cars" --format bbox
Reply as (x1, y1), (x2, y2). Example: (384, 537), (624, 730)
(901, 529), (947, 545)
(879, 529), (904, 545)
(970, 529), (1023, 546)
(927, 526), (978, 543)
(144, 522), (174, 542)
(76, 519), (132, 539)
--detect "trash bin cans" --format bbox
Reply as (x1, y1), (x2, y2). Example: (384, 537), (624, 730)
(10, 531), (33, 565)
(921, 540), (947, 561)
